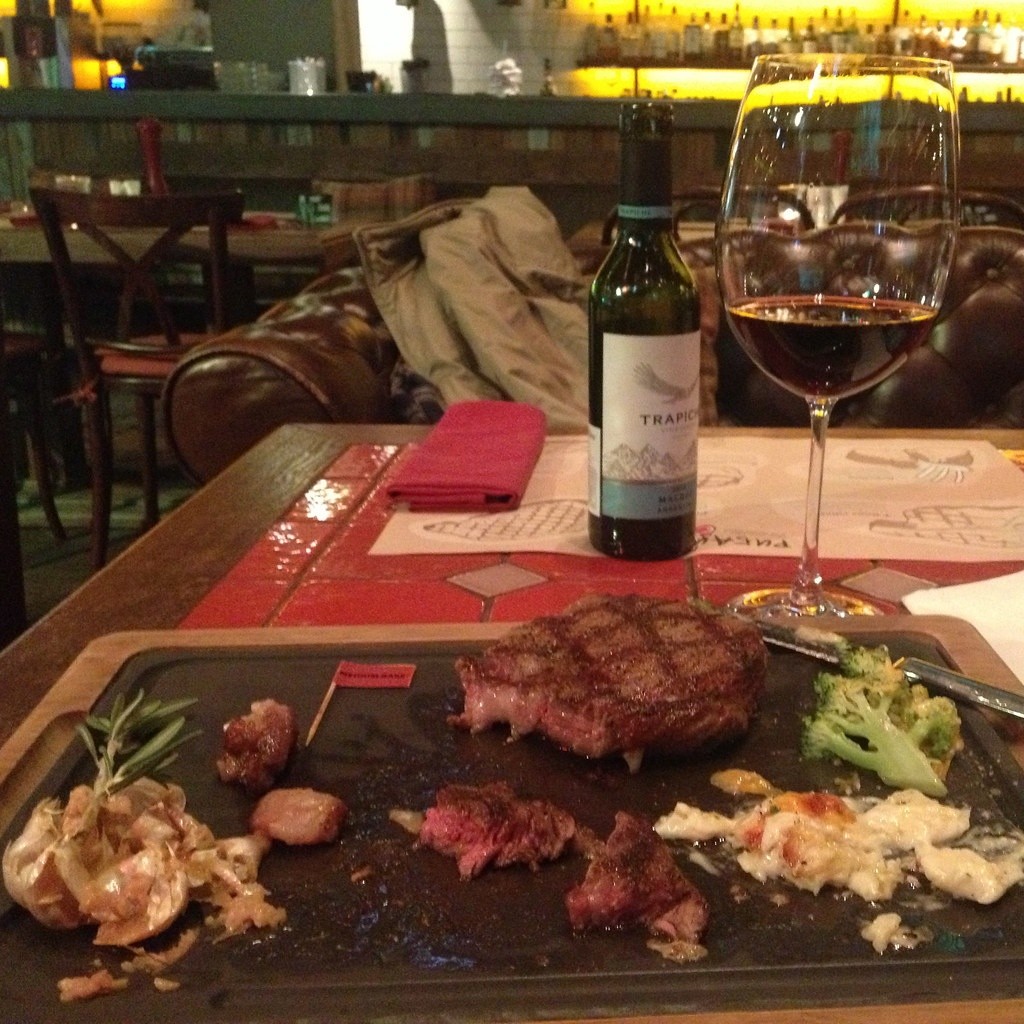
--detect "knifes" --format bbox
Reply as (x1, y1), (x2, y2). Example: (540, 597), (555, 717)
(689, 596), (1024, 719)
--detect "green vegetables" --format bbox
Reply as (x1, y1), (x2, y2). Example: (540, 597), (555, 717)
(74, 685), (207, 846)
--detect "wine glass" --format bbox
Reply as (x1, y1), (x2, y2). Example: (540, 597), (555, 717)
(715, 53), (961, 626)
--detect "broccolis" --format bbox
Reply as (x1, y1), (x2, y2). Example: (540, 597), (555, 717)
(799, 644), (961, 797)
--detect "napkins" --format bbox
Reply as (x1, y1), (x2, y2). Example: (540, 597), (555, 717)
(386, 398), (550, 514)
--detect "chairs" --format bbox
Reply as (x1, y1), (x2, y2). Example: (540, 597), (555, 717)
(154, 180), (1024, 491)
(25, 183), (249, 581)
(829, 184), (1024, 231)
(280, 171), (439, 308)
(599, 184), (815, 248)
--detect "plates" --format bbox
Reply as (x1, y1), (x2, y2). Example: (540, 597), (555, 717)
(0, 647), (1023, 1023)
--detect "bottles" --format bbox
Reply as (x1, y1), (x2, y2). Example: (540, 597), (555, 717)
(588, 100), (700, 561)
(539, 59), (558, 95)
(582, 2), (1023, 61)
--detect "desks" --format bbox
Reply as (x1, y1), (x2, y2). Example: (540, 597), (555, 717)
(0, 423), (1024, 1024)
(566, 214), (794, 276)
(0, 219), (320, 543)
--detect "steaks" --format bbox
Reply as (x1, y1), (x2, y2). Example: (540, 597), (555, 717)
(567, 809), (709, 941)
(412, 781), (581, 878)
(450, 596), (769, 756)
